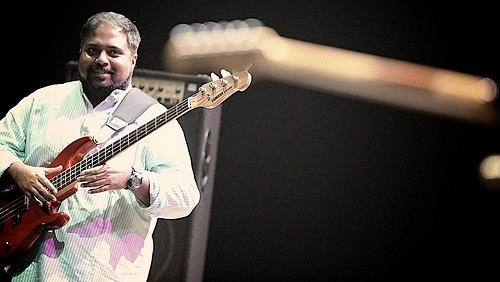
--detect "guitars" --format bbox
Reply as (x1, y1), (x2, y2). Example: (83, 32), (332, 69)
(164, 19), (500, 179)
(0, 61), (253, 266)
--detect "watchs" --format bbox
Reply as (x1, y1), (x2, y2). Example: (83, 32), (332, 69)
(123, 166), (143, 191)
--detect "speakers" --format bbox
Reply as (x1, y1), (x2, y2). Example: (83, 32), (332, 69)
(69, 63), (221, 282)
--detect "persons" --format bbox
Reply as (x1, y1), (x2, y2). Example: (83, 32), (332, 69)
(0, 12), (200, 282)
